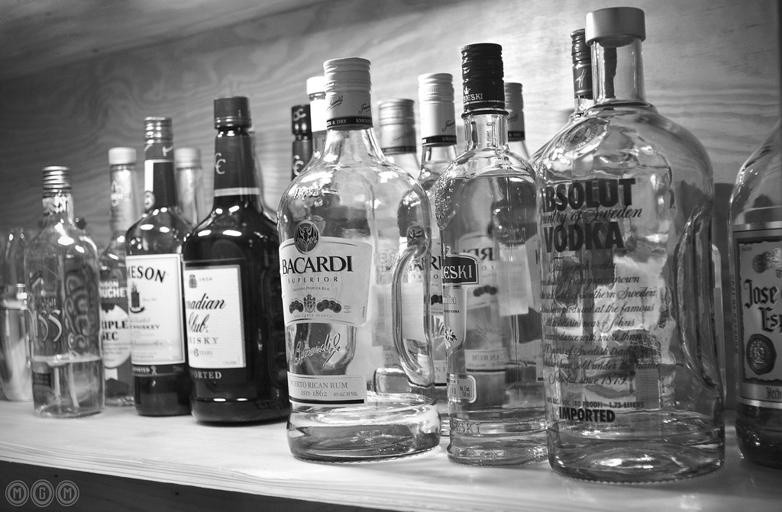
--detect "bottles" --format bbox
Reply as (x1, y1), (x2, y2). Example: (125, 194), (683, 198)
(1, 7), (782, 486)
